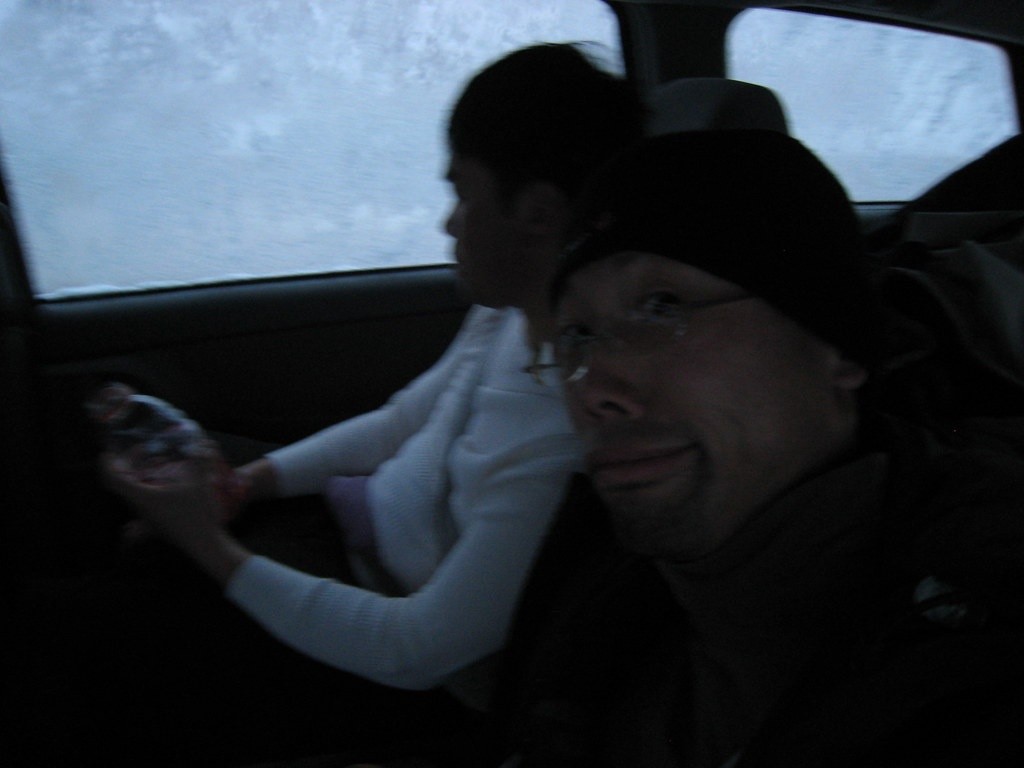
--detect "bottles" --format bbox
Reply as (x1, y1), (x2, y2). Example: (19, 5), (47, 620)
(89, 383), (258, 544)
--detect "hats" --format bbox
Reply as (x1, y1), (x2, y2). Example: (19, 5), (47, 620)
(546, 128), (862, 361)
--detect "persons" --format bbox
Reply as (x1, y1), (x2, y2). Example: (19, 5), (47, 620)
(501, 126), (1024, 768)
(97, 41), (628, 748)
